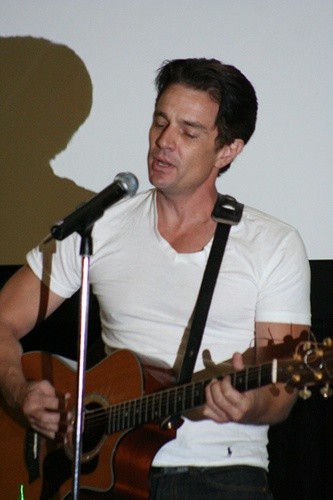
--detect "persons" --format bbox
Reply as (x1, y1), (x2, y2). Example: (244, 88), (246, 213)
(0, 59), (313, 500)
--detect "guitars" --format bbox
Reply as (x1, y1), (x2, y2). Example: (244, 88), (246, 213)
(0, 335), (333, 500)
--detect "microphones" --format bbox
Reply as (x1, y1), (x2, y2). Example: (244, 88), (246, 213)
(50, 172), (139, 242)
